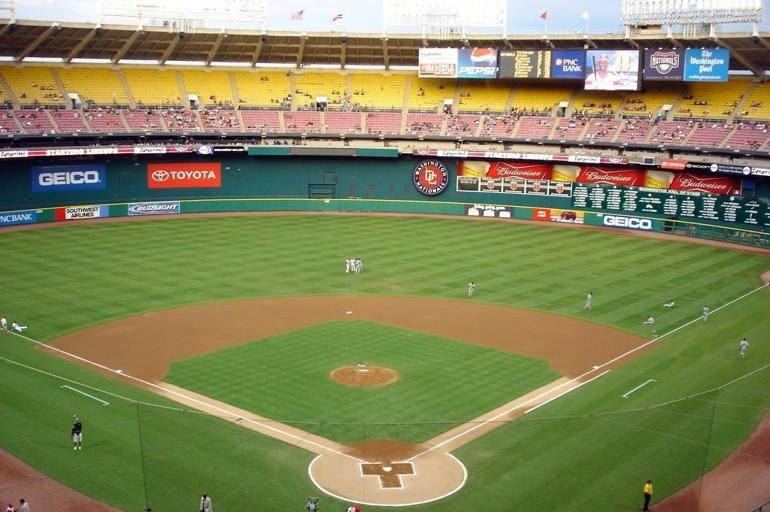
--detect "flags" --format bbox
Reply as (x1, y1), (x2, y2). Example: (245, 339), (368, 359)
(332, 13), (343, 22)
(291, 9), (304, 21)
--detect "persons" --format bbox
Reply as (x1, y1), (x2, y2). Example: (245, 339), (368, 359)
(6, 499), (15, 512)
(16, 498), (32, 511)
(584, 291), (593, 311)
(643, 315), (654, 325)
(70, 417), (83, 450)
(12, 320), (27, 332)
(642, 479), (654, 511)
(200, 491), (213, 512)
(1, 74), (770, 164)
(468, 281), (475, 296)
(304, 494), (320, 512)
(664, 300), (674, 307)
(345, 257), (363, 273)
(584, 53), (620, 90)
(703, 305), (710, 322)
(1, 315), (8, 336)
(738, 337), (749, 357)
